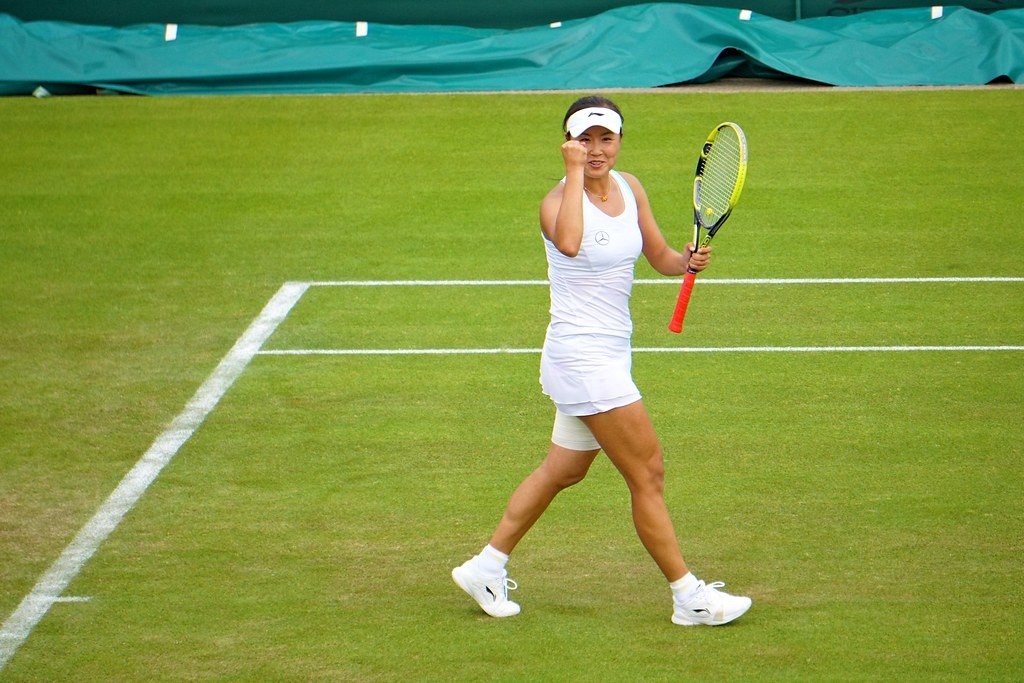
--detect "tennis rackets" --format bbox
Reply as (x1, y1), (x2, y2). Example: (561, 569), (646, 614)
(668, 121), (749, 333)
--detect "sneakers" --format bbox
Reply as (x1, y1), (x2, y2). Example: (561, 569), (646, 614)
(671, 580), (752, 626)
(452, 555), (521, 618)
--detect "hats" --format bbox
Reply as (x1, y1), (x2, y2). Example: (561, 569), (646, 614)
(566, 107), (623, 138)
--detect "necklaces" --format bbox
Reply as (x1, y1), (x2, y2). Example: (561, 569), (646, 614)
(584, 176), (611, 203)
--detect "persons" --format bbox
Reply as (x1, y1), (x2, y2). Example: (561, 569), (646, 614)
(450, 95), (754, 625)
